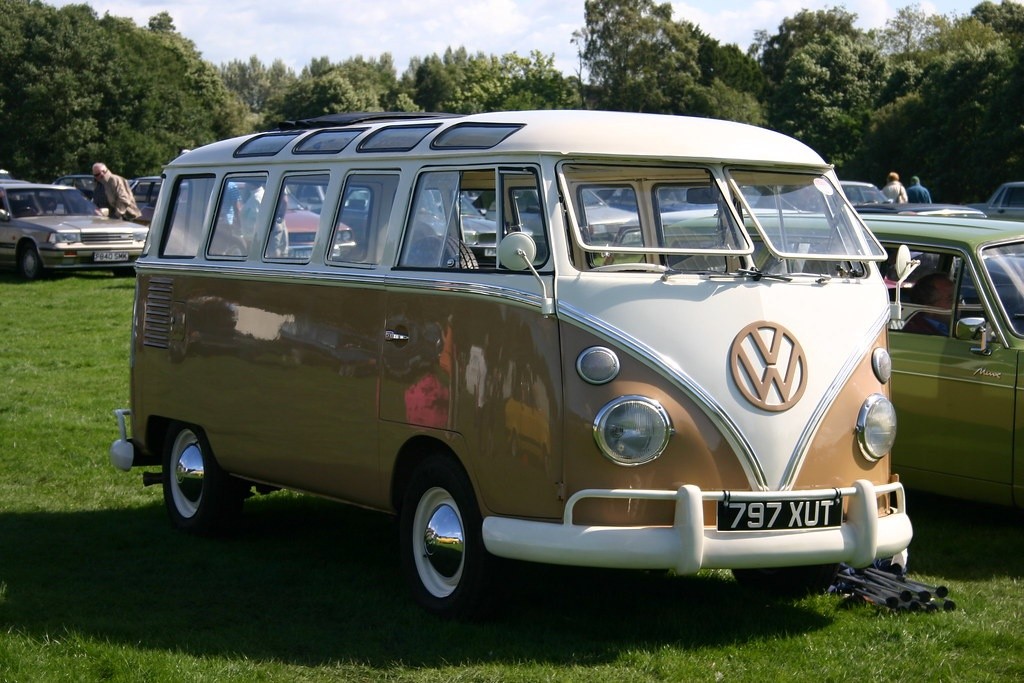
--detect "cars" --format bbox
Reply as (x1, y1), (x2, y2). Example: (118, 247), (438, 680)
(107, 112), (913, 613)
(175, 180), (762, 263)
(596, 212), (1024, 514)
(0, 165), (163, 280)
(839, 179), (1024, 220)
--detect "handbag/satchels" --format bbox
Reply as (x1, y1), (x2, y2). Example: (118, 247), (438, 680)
(897, 186), (904, 203)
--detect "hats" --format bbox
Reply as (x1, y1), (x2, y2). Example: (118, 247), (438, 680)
(911, 176), (920, 183)
(888, 172), (899, 180)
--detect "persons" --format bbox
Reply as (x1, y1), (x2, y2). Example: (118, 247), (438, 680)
(92, 163), (142, 222)
(898, 272), (962, 337)
(236, 182), (290, 259)
(882, 172), (909, 204)
(905, 176), (932, 203)
(403, 309), (554, 447)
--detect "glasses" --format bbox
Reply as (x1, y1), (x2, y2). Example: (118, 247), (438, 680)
(933, 298), (964, 305)
(94, 173), (101, 176)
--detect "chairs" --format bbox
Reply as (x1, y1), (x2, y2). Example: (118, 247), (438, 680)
(35, 197), (57, 214)
